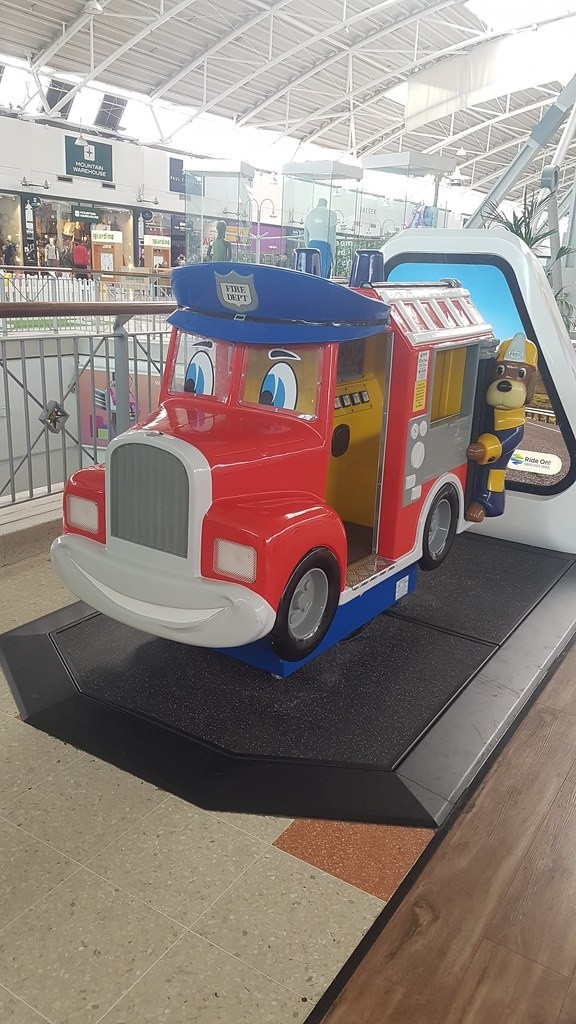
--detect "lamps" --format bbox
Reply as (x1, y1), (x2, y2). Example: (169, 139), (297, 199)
(74, 117), (89, 146)
(20, 177), (49, 190)
(342, 226), (354, 231)
(84, 0), (104, 15)
(456, 109), (467, 156)
(290, 217), (304, 224)
(136, 194), (159, 206)
(223, 206), (240, 217)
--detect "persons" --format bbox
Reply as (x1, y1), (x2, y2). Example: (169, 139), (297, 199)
(72, 246), (91, 279)
(45, 238), (59, 266)
(305, 199), (338, 278)
(209, 220), (231, 261)
(5, 236), (16, 266)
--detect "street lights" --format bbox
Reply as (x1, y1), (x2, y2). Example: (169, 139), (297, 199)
(243, 198), (277, 266)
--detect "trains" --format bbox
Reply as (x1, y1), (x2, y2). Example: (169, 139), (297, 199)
(50, 254), (537, 680)
